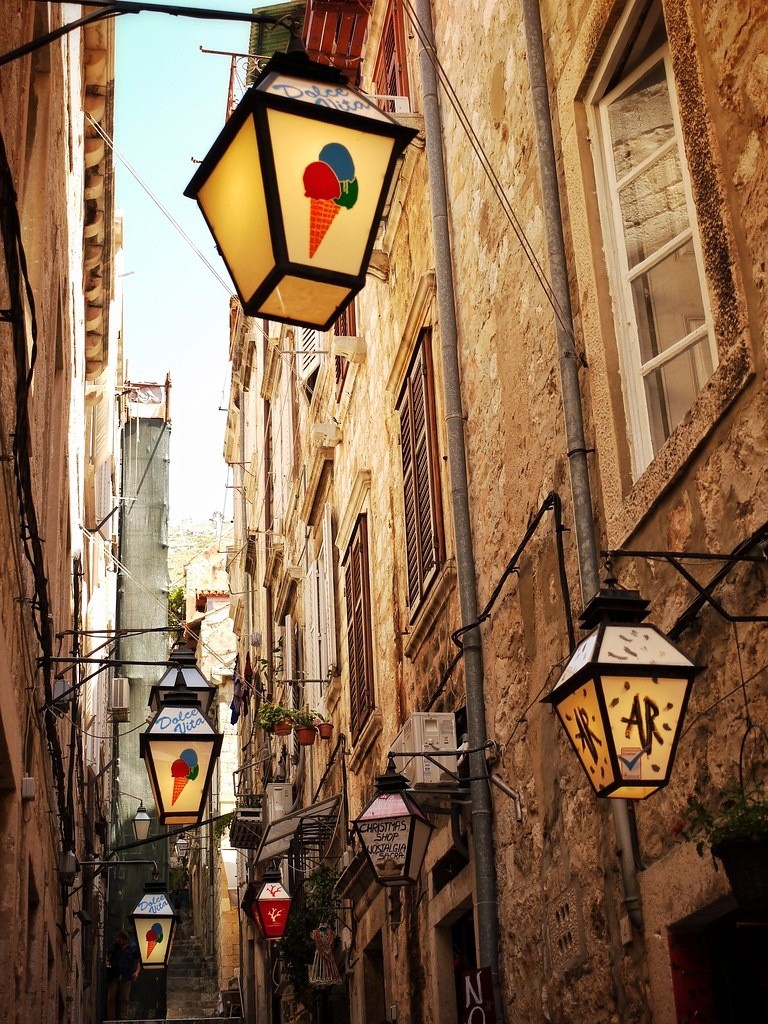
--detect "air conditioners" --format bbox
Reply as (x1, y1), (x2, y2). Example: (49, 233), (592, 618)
(388, 712), (470, 808)
(262, 783), (299, 835)
(111, 678), (130, 722)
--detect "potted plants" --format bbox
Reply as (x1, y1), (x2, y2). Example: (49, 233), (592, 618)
(289, 707), (318, 746)
(253, 701), (298, 736)
(317, 711), (334, 739)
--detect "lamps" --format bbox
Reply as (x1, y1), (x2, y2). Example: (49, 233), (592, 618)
(539, 549), (767, 801)
(58, 860), (178, 969)
(351, 741), (497, 887)
(110, 788), (153, 843)
(0, 0), (422, 332)
(250, 856), (342, 940)
(57, 627), (218, 716)
(170, 834), (189, 858)
(36, 657), (225, 825)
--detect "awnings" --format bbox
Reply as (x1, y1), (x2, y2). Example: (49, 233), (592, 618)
(252, 793), (343, 866)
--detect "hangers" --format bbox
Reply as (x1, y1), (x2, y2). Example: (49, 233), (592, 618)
(312, 910), (335, 934)
